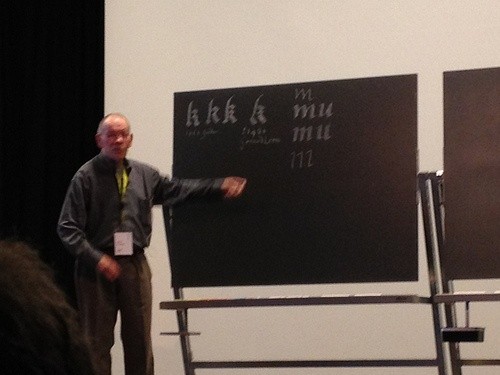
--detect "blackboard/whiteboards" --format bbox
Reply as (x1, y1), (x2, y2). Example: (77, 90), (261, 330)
(443, 67), (500, 281)
(171, 71), (419, 289)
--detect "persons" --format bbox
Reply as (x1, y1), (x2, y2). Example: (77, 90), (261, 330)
(57, 112), (247, 374)
(0, 240), (112, 374)
